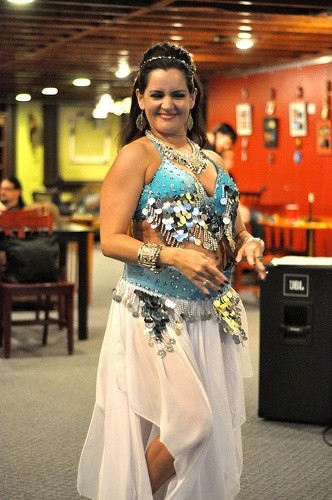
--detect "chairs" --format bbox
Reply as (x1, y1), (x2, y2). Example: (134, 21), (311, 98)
(0, 186), (312, 358)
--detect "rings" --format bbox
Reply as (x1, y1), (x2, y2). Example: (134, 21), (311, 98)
(256, 255), (262, 261)
(204, 280), (208, 285)
(207, 257), (210, 261)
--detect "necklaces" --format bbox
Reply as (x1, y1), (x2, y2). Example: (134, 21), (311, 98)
(145, 130), (208, 174)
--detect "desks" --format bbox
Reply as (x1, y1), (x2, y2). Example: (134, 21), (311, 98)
(0, 221), (97, 342)
(257, 213), (332, 258)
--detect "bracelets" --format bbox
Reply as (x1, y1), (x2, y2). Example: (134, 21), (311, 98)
(246, 237), (265, 252)
(137, 242), (168, 273)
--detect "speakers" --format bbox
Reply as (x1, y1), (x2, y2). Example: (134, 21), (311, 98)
(257, 264), (332, 426)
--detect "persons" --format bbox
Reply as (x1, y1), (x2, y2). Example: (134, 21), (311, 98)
(77, 42), (266, 500)
(206, 123), (236, 179)
(0, 174), (25, 210)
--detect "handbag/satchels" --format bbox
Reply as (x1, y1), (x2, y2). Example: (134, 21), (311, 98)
(4, 234), (60, 284)
